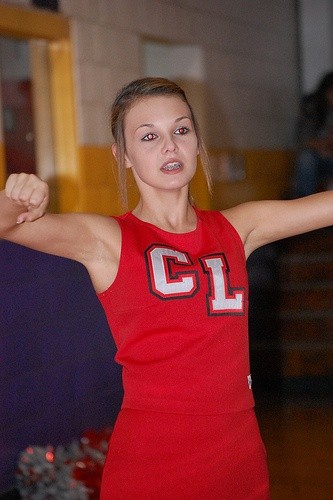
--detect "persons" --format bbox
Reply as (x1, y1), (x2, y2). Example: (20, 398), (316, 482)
(0, 76), (333, 500)
(290, 68), (332, 200)
